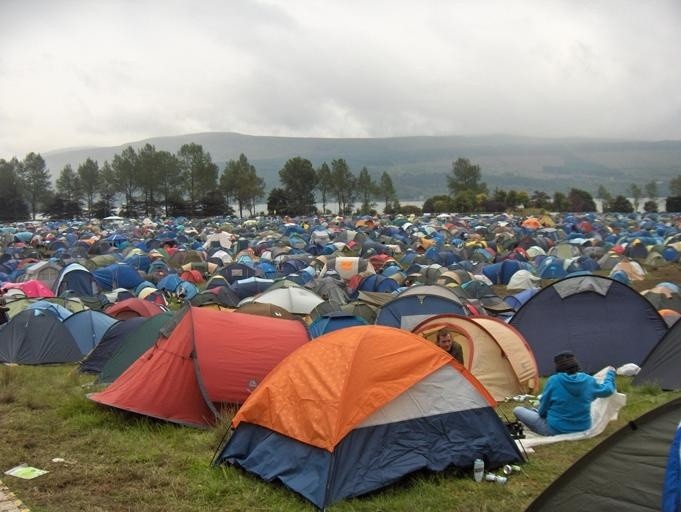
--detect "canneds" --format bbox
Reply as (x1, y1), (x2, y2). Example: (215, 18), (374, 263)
(503, 464), (522, 475)
(503, 394), (542, 406)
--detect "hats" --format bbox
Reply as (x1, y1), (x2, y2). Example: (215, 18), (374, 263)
(553, 350), (582, 374)
